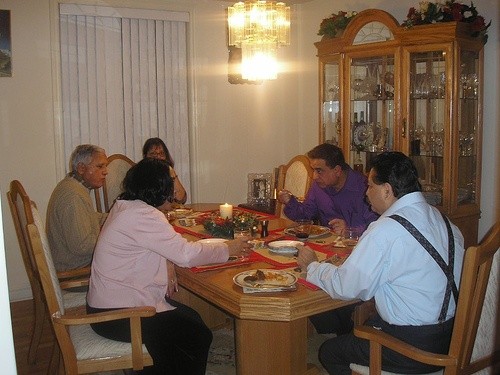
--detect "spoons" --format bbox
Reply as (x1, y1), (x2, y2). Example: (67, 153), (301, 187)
(277, 190), (308, 201)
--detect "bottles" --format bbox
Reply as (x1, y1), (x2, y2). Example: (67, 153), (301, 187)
(261, 220), (270, 238)
(352, 112), (359, 133)
(359, 110), (366, 125)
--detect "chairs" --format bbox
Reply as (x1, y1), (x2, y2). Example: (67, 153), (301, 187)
(349, 222), (500, 375)
(6, 154), (155, 375)
(274, 154), (312, 218)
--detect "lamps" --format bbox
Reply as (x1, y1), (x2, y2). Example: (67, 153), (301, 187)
(226, 0), (292, 81)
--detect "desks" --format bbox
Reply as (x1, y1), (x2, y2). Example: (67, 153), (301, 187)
(164, 205), (364, 375)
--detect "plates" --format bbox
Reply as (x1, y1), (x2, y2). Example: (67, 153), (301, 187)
(195, 238), (229, 243)
(233, 268), (299, 290)
(175, 209), (191, 214)
(284, 225), (331, 238)
(319, 253), (350, 267)
(267, 240), (305, 253)
(353, 125), (374, 148)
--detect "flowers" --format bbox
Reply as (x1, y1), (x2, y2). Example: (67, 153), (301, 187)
(402, 0), (492, 44)
(317, 9), (357, 35)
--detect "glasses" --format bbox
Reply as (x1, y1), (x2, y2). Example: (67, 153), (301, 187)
(148, 150), (167, 157)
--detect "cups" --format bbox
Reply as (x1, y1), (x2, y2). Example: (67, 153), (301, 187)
(296, 218), (314, 241)
(166, 205), (177, 221)
(233, 227), (251, 259)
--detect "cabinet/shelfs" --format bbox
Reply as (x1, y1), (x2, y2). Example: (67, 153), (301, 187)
(314, 11), (482, 244)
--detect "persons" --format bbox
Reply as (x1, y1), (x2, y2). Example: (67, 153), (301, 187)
(143, 137), (188, 205)
(87, 157), (253, 375)
(278, 143), (380, 334)
(45, 145), (109, 293)
(296, 151), (465, 375)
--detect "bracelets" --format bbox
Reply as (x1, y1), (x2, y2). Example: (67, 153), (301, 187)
(171, 176), (177, 179)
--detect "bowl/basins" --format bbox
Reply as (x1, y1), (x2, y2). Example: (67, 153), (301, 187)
(418, 181), (475, 208)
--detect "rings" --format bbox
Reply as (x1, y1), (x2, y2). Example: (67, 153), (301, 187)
(171, 279), (174, 283)
(243, 249), (245, 251)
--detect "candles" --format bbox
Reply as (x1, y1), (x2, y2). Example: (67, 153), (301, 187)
(219, 203), (233, 221)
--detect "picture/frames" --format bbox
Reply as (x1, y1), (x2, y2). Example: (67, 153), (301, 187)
(0, 9), (12, 77)
(249, 172), (271, 207)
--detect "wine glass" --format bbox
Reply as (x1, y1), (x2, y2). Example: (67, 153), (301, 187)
(324, 68), (478, 156)
(340, 228), (359, 259)
